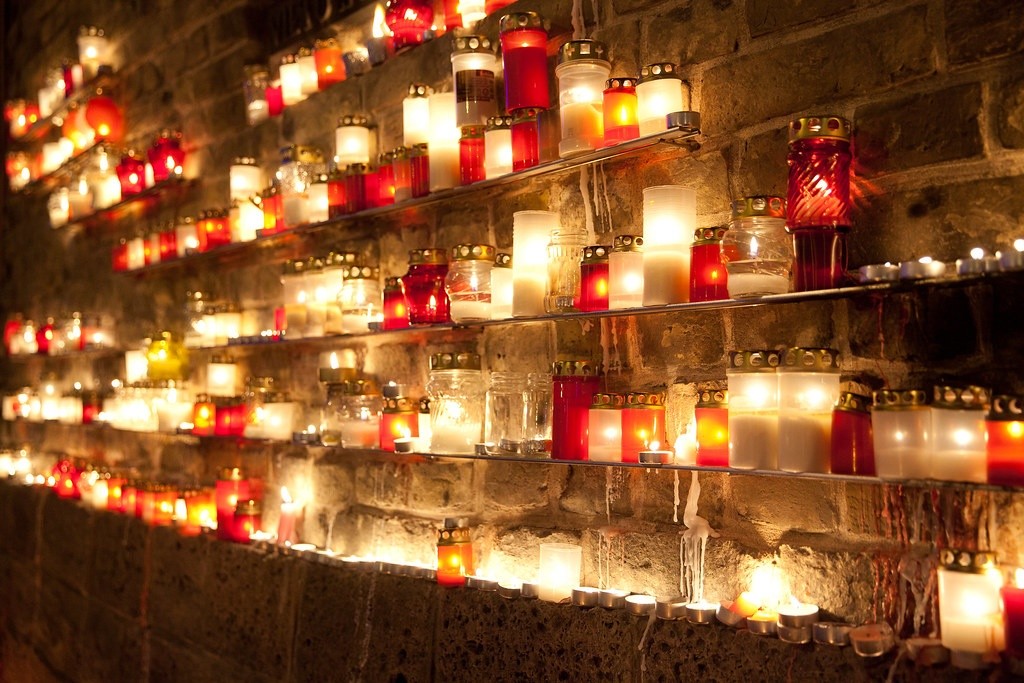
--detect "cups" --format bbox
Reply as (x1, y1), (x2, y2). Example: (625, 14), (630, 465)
(0, 0), (1024, 653)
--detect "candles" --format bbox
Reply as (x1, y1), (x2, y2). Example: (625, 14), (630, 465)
(2, 1), (1024, 680)
